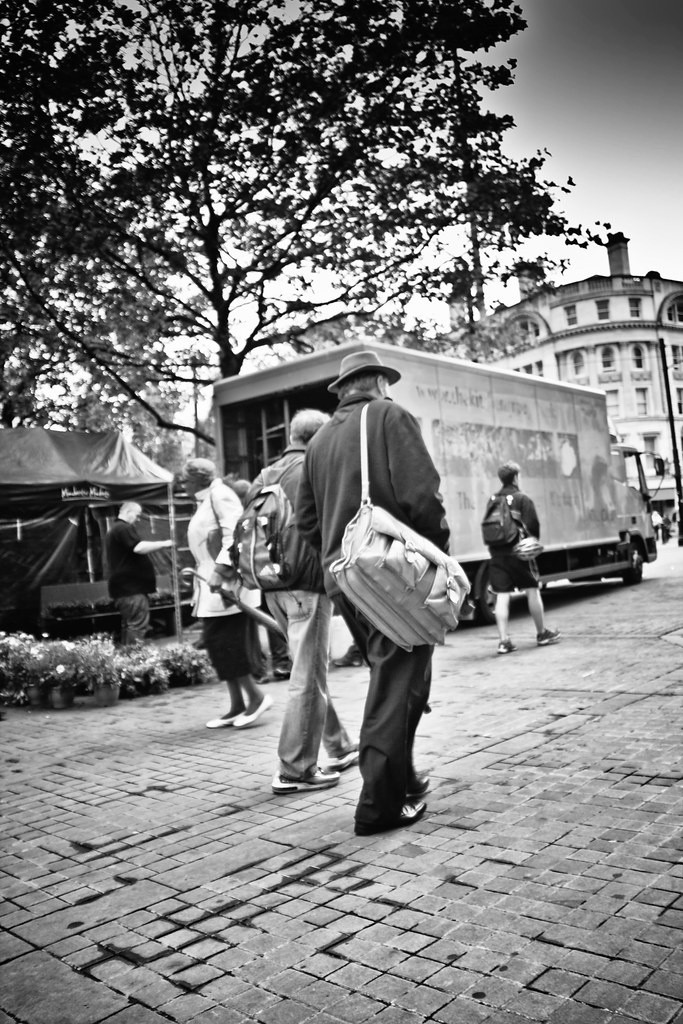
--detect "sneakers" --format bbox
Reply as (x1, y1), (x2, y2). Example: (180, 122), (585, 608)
(329, 737), (361, 769)
(273, 767), (340, 794)
(536, 629), (560, 644)
(498, 641), (517, 654)
(332, 656), (362, 665)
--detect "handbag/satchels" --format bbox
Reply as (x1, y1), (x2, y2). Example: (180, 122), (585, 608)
(328, 504), (470, 652)
(208, 496), (237, 577)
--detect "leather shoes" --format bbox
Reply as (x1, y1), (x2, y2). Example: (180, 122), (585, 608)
(355, 800), (426, 836)
(405, 776), (430, 795)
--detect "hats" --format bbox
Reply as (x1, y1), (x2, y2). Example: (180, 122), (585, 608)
(327, 350), (400, 394)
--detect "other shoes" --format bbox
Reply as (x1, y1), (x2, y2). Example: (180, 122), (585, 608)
(234, 696), (274, 727)
(205, 710), (248, 728)
(274, 666), (290, 678)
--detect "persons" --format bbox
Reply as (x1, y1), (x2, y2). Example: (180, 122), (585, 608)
(217, 474), (363, 686)
(291, 348), (456, 837)
(103, 502), (177, 648)
(650, 510), (673, 543)
(486, 461), (561, 655)
(180, 456), (275, 728)
(249, 407), (359, 795)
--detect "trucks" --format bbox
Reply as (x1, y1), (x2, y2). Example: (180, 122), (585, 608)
(211, 334), (666, 625)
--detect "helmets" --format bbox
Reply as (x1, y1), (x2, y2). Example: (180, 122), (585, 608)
(513, 536), (542, 561)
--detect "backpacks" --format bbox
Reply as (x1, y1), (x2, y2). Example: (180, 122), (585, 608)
(230, 456), (311, 590)
(480, 489), (518, 545)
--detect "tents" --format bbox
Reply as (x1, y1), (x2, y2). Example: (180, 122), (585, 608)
(0, 425), (181, 641)
